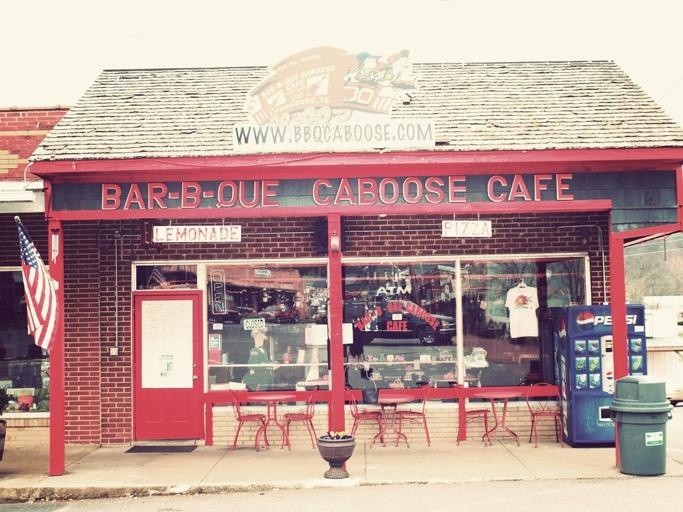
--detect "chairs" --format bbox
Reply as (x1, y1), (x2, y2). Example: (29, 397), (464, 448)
(344, 384), (390, 446)
(519, 381), (568, 452)
(453, 382), (499, 447)
(395, 383), (432, 450)
(228, 390), (269, 451)
(281, 386), (321, 454)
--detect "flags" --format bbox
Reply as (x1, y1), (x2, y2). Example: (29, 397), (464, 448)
(16, 218), (59, 353)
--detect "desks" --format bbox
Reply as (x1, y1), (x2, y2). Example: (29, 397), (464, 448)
(469, 386), (528, 447)
(369, 389), (415, 452)
(241, 393), (301, 454)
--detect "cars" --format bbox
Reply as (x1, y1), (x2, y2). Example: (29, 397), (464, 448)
(207, 299), (454, 376)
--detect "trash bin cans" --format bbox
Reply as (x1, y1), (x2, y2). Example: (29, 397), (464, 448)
(609, 376), (674, 476)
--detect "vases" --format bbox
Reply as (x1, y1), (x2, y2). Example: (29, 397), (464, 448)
(317, 435), (356, 480)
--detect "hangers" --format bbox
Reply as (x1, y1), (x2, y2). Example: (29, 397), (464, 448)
(513, 277), (530, 289)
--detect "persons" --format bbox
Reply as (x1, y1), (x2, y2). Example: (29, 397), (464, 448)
(241, 327), (281, 391)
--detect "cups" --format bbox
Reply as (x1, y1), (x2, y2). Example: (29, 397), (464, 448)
(283, 353), (289, 363)
(221, 353), (228, 364)
(298, 349), (303, 362)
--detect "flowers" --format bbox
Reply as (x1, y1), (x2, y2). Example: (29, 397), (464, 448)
(324, 428), (346, 440)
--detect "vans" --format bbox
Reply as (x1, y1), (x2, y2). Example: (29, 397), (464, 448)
(473, 300), (505, 339)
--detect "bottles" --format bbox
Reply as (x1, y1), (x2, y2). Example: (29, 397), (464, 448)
(286, 345), (293, 363)
